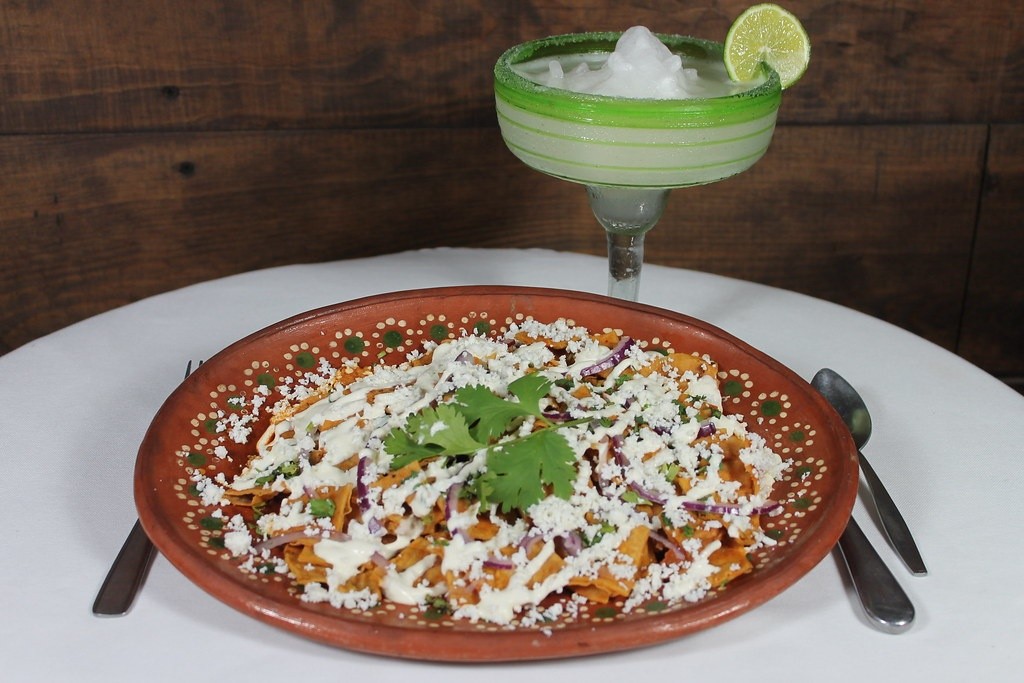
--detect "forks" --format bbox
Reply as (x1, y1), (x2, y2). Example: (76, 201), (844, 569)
(91, 359), (209, 617)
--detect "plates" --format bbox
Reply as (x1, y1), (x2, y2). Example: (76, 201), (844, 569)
(133, 284), (860, 663)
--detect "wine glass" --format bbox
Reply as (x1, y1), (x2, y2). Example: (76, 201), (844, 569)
(493, 30), (782, 305)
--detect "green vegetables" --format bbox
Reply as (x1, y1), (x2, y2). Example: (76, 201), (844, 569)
(385, 369), (596, 513)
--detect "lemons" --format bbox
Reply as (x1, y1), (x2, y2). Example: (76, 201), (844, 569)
(723, 3), (812, 91)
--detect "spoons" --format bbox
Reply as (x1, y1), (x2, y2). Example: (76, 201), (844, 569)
(810, 367), (928, 577)
